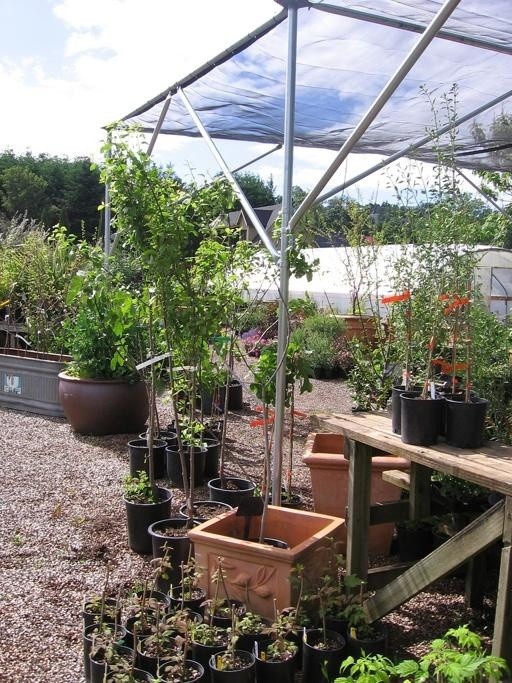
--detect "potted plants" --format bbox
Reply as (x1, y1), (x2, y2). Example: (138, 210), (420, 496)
(3, 81), (511, 683)
